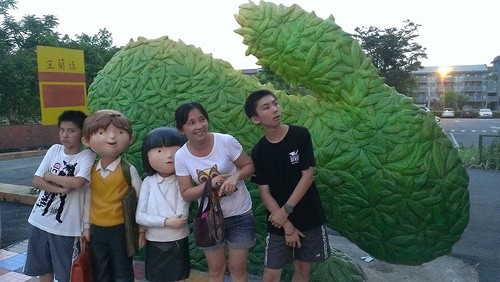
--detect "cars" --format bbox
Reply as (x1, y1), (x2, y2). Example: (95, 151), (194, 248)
(476, 109), (493, 119)
(442, 108), (454, 118)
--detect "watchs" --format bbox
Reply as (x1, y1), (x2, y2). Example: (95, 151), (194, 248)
(284, 204), (294, 213)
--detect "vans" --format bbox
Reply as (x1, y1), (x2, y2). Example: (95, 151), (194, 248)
(462, 105), (473, 117)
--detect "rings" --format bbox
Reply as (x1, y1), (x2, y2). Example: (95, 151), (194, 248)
(215, 182), (219, 185)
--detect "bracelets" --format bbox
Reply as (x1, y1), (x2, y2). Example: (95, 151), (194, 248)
(285, 227), (296, 237)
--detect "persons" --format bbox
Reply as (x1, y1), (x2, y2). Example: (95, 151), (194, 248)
(80, 109), (145, 282)
(135, 126), (193, 282)
(23, 89), (330, 282)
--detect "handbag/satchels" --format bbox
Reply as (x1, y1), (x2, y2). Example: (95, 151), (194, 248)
(195, 178), (225, 247)
